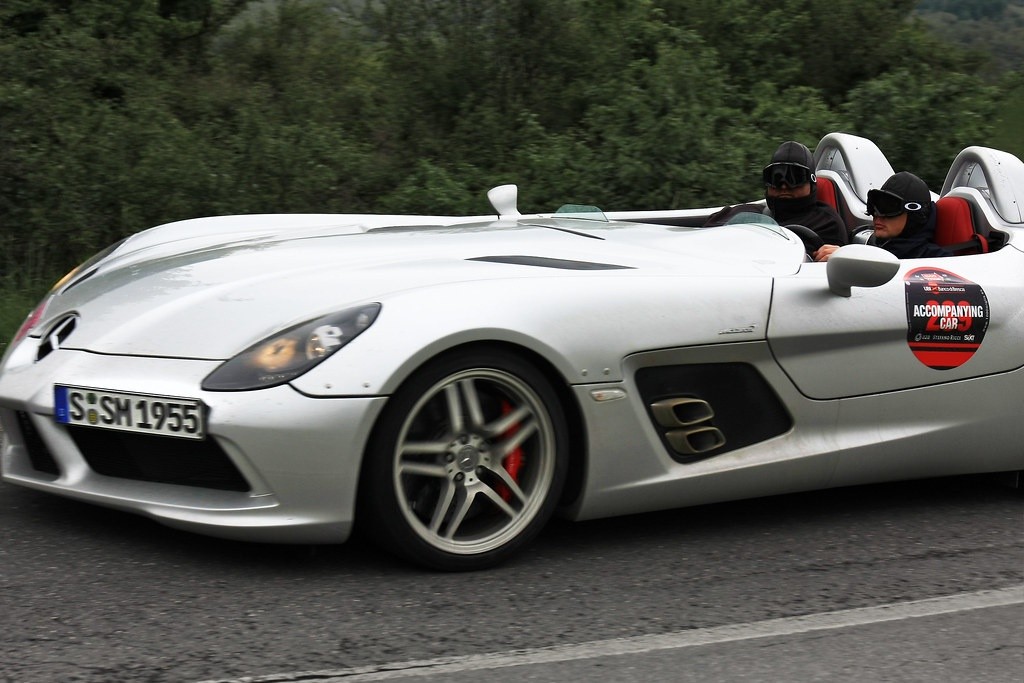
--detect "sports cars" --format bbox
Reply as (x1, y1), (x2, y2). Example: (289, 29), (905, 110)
(0, 132), (1024, 575)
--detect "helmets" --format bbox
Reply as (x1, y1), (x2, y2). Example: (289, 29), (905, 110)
(880, 172), (931, 203)
(771, 141), (814, 174)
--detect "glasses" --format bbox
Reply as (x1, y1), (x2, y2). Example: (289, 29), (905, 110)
(867, 189), (905, 217)
(762, 162), (810, 189)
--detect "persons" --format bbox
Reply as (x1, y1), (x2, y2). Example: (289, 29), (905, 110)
(761, 141), (850, 262)
(812, 171), (962, 262)
